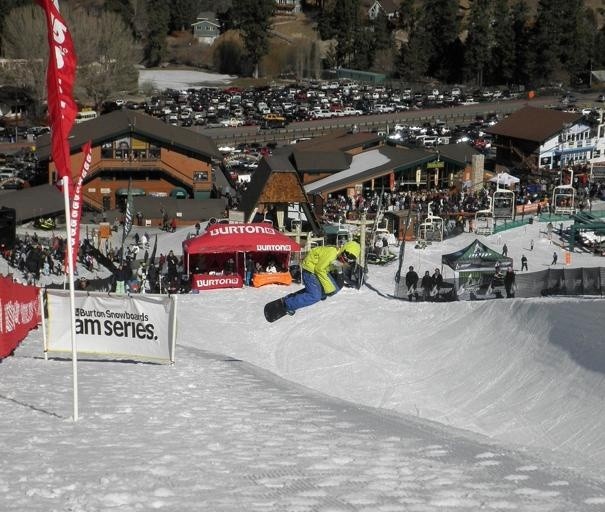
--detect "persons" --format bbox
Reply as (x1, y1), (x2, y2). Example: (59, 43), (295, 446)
(281, 240), (360, 315)
(1, 210), (281, 293)
(323, 182), (605, 301)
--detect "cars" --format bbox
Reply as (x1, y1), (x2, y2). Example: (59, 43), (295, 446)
(0, 107), (101, 196)
(543, 78), (605, 118)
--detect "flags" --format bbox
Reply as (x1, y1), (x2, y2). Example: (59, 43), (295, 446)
(32, 0), (79, 198)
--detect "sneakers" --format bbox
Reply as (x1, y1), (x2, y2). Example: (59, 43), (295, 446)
(282, 296), (295, 316)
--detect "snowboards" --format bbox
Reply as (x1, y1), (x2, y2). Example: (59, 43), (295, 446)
(264, 272), (344, 323)
